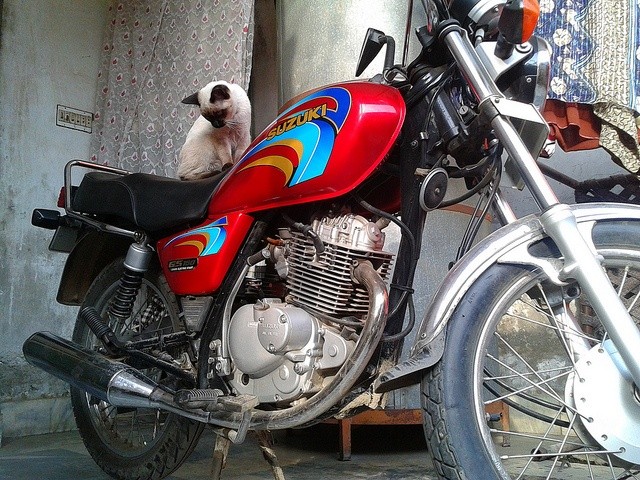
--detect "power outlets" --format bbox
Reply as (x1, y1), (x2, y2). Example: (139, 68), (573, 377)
(56, 103), (93, 135)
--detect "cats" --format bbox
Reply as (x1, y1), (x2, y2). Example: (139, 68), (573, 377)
(175, 81), (252, 182)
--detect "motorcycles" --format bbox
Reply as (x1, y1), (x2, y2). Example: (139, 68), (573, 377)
(22, 1), (639, 475)
